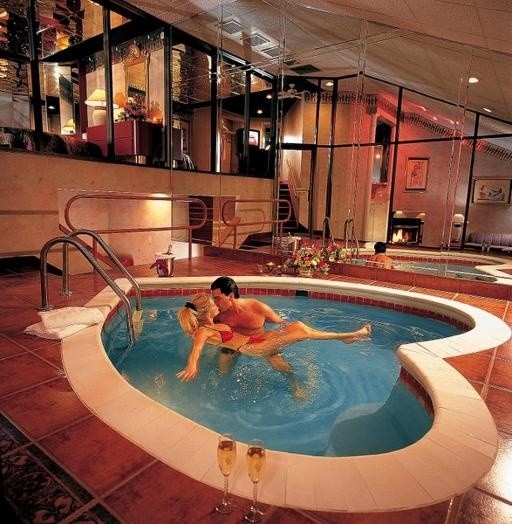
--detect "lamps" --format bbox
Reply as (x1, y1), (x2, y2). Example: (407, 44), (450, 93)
(84, 89), (119, 126)
(62, 119), (75, 135)
(449, 213), (470, 241)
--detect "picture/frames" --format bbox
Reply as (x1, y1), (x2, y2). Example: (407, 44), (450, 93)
(472, 177), (512, 204)
(172, 118), (190, 156)
(405, 156), (429, 192)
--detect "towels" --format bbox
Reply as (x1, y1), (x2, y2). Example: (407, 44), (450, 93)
(23, 306), (105, 340)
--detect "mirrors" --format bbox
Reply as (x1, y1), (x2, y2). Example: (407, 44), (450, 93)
(121, 53), (150, 113)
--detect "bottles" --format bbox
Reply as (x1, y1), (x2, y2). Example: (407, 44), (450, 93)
(165, 243), (173, 254)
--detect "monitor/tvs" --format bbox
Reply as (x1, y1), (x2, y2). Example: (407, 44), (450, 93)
(237, 128), (260, 153)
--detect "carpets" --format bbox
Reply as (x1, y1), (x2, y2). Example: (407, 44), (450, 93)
(0, 409), (126, 524)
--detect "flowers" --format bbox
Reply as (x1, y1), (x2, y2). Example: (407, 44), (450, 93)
(284, 247), (331, 275)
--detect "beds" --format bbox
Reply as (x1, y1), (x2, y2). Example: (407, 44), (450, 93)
(0, 127), (104, 162)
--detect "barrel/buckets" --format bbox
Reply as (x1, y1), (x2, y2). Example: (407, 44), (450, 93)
(287, 232), (302, 256)
(149, 252), (176, 277)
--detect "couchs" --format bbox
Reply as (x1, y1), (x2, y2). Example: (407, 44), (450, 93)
(464, 232), (512, 252)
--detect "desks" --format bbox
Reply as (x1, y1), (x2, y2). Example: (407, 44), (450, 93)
(172, 127), (184, 168)
(86, 120), (163, 164)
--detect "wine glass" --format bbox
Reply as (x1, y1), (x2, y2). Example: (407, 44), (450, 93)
(215, 432), (267, 519)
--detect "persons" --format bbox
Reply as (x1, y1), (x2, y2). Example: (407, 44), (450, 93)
(176, 276), (372, 399)
(366, 242), (392, 269)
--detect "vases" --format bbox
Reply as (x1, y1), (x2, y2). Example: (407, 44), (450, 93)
(298, 268), (314, 277)
